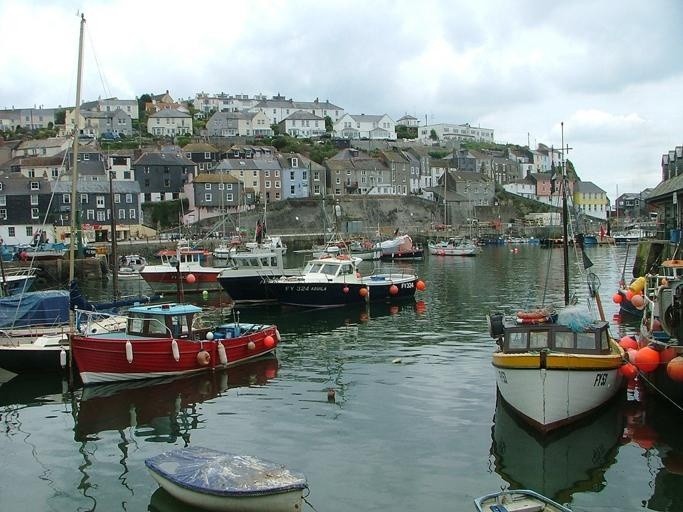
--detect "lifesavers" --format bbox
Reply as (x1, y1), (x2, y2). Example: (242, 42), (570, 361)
(518, 313), (548, 323)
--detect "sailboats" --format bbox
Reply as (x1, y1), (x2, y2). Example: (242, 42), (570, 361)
(487, 115), (624, 435)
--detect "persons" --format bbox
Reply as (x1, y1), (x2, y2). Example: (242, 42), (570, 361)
(356, 267), (362, 280)
(256, 223), (266, 245)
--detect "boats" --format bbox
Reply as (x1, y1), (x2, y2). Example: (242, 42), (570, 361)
(500, 229), (655, 246)
(615, 258), (683, 414)
(147, 445), (310, 512)
(428, 236), (485, 256)
